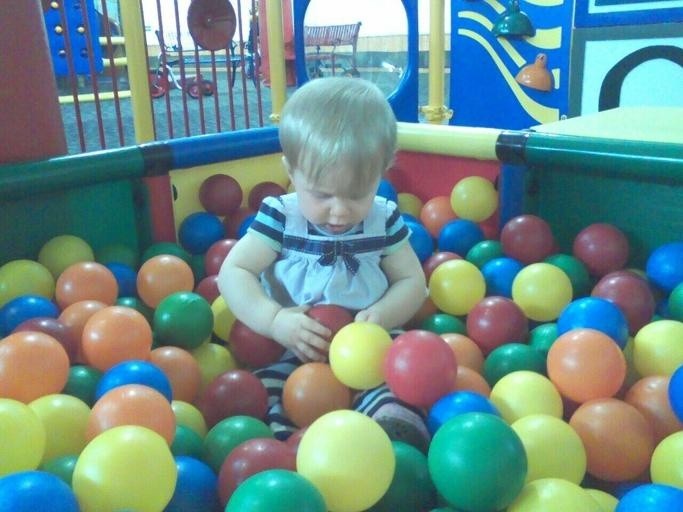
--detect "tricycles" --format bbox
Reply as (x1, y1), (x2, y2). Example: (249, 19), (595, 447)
(147, 29), (215, 99)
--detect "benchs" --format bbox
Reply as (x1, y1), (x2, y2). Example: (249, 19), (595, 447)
(154, 23), (260, 88)
(304, 21), (361, 78)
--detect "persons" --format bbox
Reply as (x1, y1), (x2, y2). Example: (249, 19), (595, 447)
(213, 75), (434, 455)
